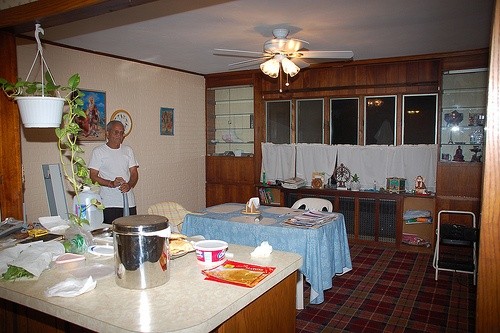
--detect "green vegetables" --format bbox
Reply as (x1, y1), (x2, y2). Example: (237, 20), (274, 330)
(2, 264), (35, 283)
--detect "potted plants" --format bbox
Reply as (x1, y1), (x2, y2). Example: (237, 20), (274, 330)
(0, 24), (103, 227)
(350, 173), (360, 191)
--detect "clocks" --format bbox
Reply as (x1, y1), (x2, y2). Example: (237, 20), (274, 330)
(110, 110), (132, 137)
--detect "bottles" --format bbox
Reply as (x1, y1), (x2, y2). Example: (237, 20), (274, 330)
(73, 186), (103, 229)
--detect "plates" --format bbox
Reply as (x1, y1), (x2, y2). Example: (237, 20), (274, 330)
(454, 141), (466, 144)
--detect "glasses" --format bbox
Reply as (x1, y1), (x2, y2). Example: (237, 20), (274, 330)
(109, 131), (124, 135)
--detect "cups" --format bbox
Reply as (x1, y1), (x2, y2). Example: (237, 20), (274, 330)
(442, 153), (452, 162)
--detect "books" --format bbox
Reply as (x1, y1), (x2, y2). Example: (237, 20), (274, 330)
(282, 177), (306, 189)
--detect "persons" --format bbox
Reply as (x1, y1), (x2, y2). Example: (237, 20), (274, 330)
(87, 120), (139, 223)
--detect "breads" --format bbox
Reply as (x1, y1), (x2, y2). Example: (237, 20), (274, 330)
(170, 233), (196, 255)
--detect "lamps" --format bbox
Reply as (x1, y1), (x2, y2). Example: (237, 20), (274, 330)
(260, 54), (300, 92)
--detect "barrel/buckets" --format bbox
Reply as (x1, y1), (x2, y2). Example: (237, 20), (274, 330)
(112, 215), (171, 290)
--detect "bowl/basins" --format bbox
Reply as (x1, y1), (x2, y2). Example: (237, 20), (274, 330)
(194, 239), (228, 265)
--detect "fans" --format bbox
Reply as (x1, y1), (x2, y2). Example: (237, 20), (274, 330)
(216, 29), (353, 69)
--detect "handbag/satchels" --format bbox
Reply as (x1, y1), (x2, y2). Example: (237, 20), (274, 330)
(63, 220), (94, 254)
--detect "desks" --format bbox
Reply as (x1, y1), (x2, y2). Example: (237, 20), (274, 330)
(0, 222), (303, 333)
(181, 203), (352, 305)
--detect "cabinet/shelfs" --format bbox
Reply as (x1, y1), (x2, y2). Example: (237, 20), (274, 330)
(436, 71), (488, 264)
(255, 182), (435, 253)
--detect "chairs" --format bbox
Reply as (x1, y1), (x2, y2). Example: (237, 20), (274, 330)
(433, 210), (477, 285)
(148, 201), (190, 233)
(291, 198), (333, 212)
